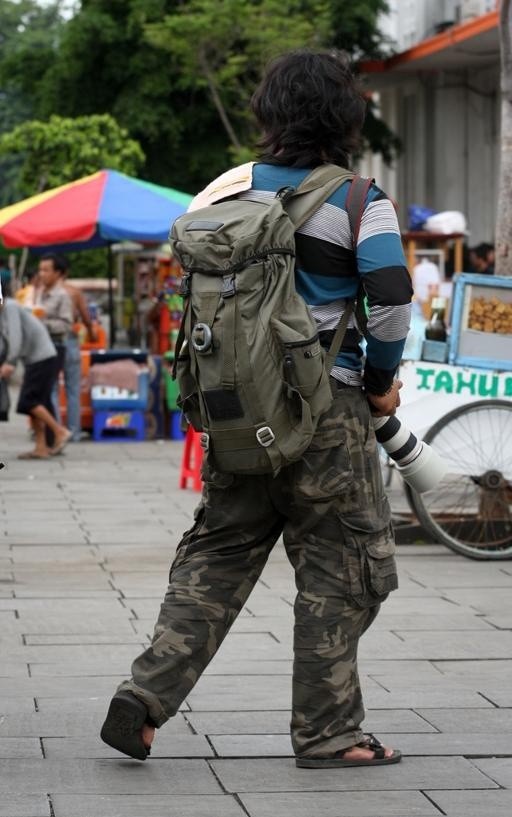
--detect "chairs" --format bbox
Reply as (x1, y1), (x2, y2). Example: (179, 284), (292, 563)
(90, 369), (149, 443)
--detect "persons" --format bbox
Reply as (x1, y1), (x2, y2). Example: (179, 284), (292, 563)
(1, 251), (100, 460)
(96, 48), (419, 768)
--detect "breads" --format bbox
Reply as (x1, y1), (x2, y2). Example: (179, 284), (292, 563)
(470, 297), (512, 333)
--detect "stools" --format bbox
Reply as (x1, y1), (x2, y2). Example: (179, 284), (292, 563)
(179, 423), (204, 492)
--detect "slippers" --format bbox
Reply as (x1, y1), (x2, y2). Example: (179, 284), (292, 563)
(50, 432), (73, 455)
(102, 693), (153, 762)
(296, 733), (404, 771)
(17, 451), (51, 460)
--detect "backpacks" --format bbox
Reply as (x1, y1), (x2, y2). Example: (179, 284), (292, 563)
(167, 159), (357, 489)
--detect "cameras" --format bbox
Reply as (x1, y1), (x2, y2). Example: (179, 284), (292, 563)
(362, 387), (444, 495)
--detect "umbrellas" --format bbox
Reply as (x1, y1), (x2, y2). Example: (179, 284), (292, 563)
(0, 166), (197, 348)
